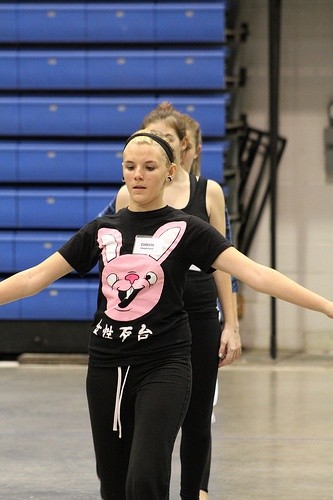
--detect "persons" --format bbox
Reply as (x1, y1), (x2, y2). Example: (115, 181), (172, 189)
(95, 113), (238, 498)
(115, 103), (243, 500)
(0, 128), (333, 499)
